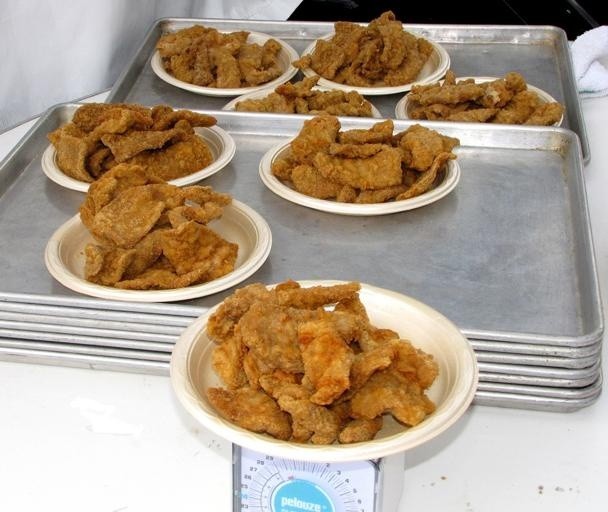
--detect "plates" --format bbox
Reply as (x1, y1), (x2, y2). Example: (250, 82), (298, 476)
(258, 138), (461, 215)
(221, 85), (382, 119)
(40, 124), (237, 192)
(394, 76), (564, 127)
(151, 29), (300, 97)
(300, 32), (451, 96)
(44, 194), (272, 303)
(168, 280), (479, 464)
